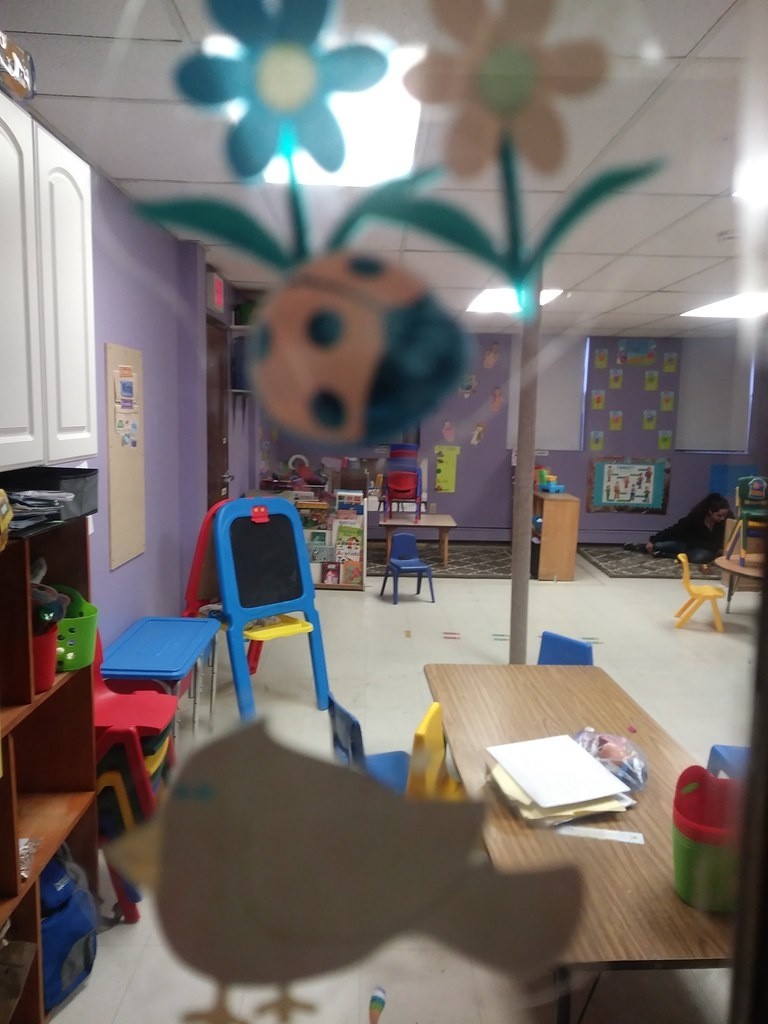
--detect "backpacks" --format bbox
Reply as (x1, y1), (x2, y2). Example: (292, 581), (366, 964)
(39, 857), (100, 1017)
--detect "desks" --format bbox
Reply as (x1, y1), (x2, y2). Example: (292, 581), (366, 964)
(715, 554), (765, 614)
(378, 513), (458, 568)
(423, 663), (739, 1024)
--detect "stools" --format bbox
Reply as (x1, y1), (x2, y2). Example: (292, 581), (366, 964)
(706, 745), (748, 781)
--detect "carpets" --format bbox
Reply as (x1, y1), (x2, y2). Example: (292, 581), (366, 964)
(578, 546), (721, 579)
(365, 542), (532, 579)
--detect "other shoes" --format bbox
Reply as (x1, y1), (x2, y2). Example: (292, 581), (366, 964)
(624, 541), (640, 552)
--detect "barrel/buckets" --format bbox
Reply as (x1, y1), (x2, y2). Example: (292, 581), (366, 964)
(673, 763), (737, 915)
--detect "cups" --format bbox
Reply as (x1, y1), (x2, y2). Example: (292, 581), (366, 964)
(30, 626), (59, 693)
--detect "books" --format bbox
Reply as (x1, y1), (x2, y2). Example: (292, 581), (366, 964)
(310, 491), (364, 584)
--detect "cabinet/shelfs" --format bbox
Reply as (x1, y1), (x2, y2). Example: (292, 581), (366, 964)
(0, 518), (104, 1024)
(511, 483), (581, 580)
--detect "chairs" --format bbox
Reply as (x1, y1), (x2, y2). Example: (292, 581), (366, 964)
(380, 533), (435, 604)
(726, 477), (768, 564)
(90, 635), (177, 832)
(538, 632), (592, 665)
(379, 442), (422, 524)
(326, 691), (411, 791)
(406, 702), (464, 800)
(675, 553), (725, 632)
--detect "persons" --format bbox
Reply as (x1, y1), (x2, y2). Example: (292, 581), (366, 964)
(624, 493), (734, 563)
